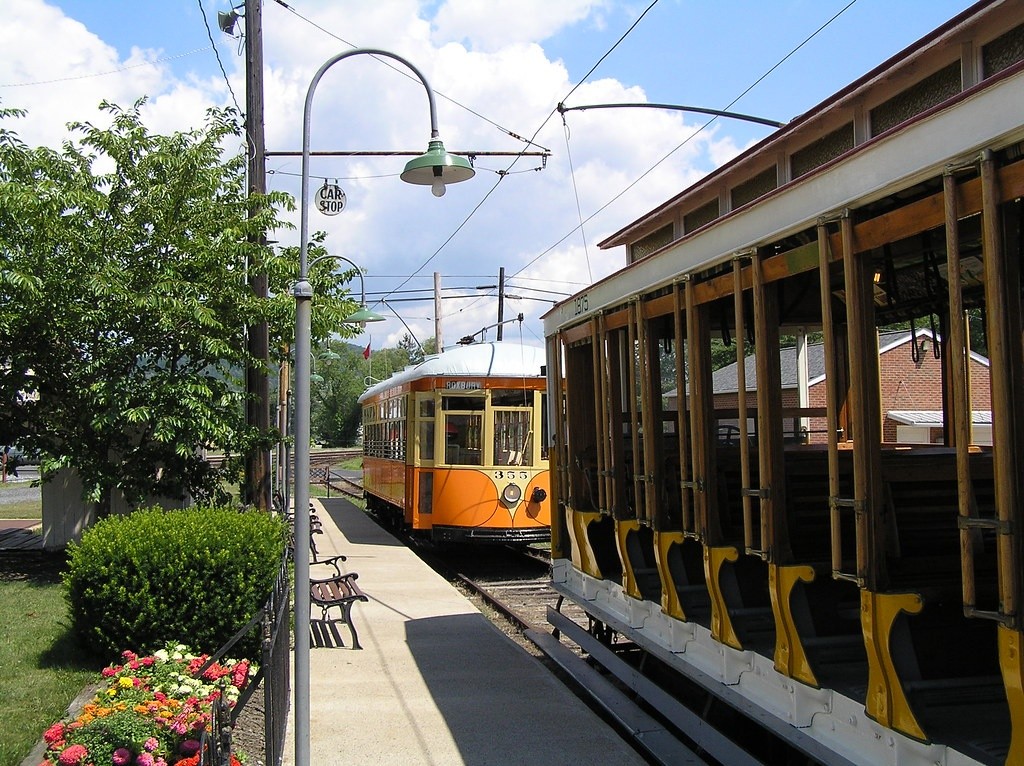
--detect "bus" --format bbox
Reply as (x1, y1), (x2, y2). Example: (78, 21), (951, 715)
(539, 1), (1024, 765)
(356, 313), (568, 549)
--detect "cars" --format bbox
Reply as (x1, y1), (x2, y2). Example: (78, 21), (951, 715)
(0, 444), (44, 465)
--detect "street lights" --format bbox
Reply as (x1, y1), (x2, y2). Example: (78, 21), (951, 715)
(294, 47), (478, 766)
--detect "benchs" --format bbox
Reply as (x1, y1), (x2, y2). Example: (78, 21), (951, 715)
(561, 424), (1024, 766)
(273, 489), (369, 651)
